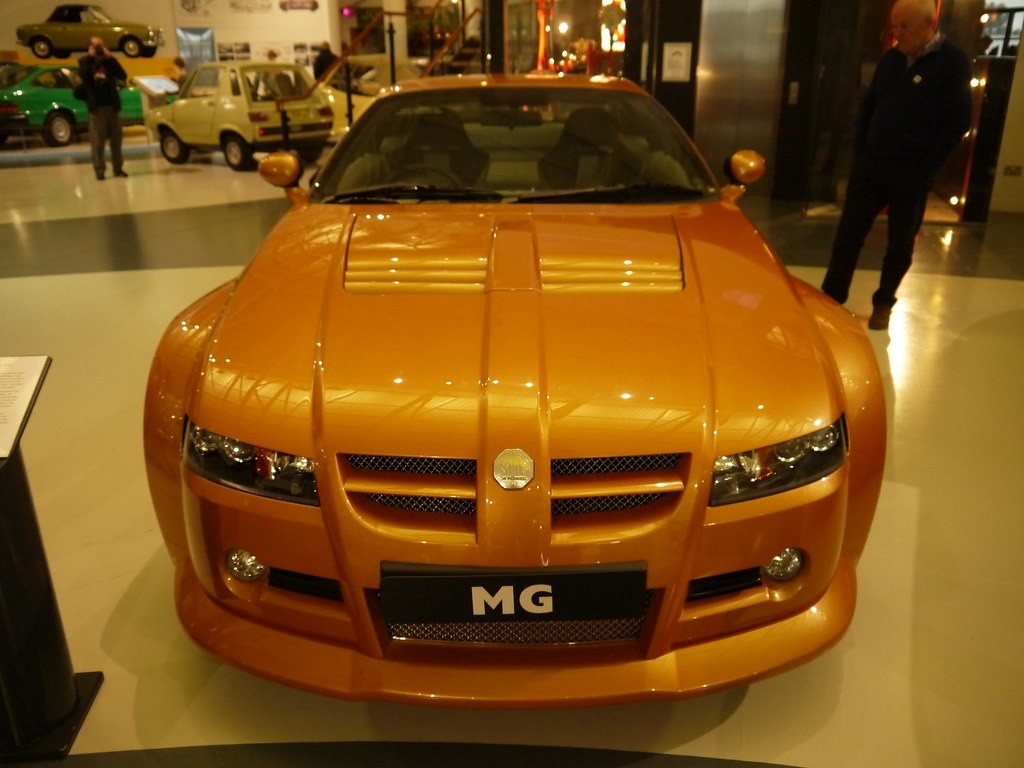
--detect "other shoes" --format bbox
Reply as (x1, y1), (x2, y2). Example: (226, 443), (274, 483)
(96, 172), (104, 180)
(115, 170), (128, 177)
(868, 305), (891, 330)
(822, 290), (848, 304)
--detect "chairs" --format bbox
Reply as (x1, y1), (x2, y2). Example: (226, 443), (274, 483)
(394, 107), (491, 187)
(536, 107), (644, 191)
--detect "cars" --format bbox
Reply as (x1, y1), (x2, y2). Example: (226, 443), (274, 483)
(144, 74), (885, 706)
(145, 61), (333, 171)
(16, 4), (164, 59)
(0, 63), (176, 148)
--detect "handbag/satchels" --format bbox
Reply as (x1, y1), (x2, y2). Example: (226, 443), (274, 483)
(74, 84), (84, 99)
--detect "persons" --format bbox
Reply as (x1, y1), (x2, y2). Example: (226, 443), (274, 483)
(78, 36), (128, 180)
(314, 43), (339, 80)
(821, 1), (973, 330)
